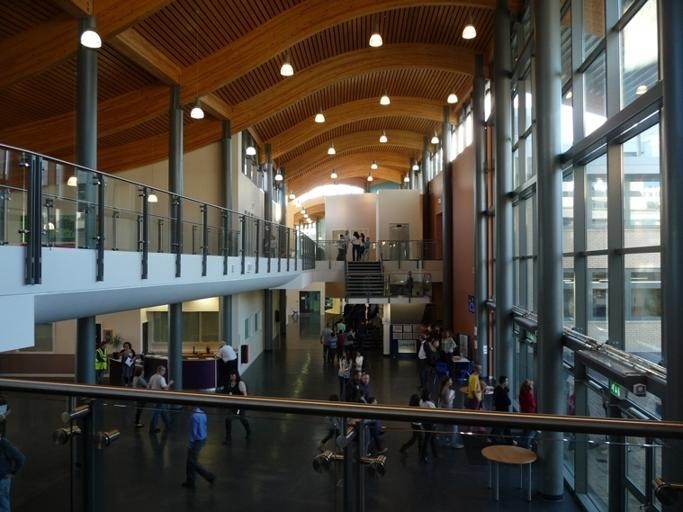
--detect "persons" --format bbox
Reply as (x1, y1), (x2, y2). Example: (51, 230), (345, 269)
(117, 342), (144, 386)
(215, 341), (238, 392)
(182, 405), (214, 487)
(317, 305), (537, 462)
(0, 394), (12, 421)
(95, 341), (109, 383)
(336, 229), (371, 261)
(407, 270), (414, 296)
(146, 365), (174, 433)
(223, 369), (251, 443)
(0, 422), (26, 512)
(131, 366), (147, 428)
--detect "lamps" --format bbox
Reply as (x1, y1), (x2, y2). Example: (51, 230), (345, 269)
(279, 53), (294, 76)
(403, 172), (410, 183)
(380, 86), (391, 104)
(80, 14), (102, 48)
(411, 159), (420, 171)
(327, 140), (336, 155)
(368, 19), (384, 47)
(189, 98), (205, 119)
(378, 129), (388, 143)
(430, 127), (440, 144)
(274, 167), (284, 181)
(314, 103), (326, 123)
(447, 87), (459, 104)
(367, 172), (374, 182)
(462, 13), (477, 39)
(330, 169), (338, 179)
(244, 136), (257, 156)
(369, 160), (378, 169)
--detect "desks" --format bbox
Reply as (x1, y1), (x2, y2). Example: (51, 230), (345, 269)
(458, 382), (495, 408)
(479, 441), (539, 505)
